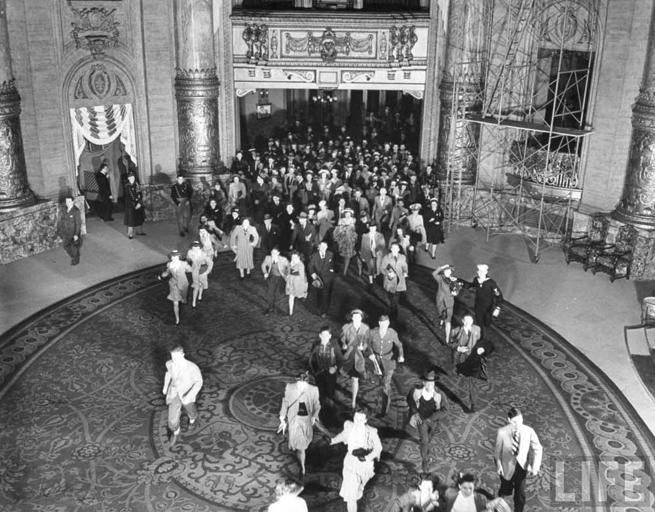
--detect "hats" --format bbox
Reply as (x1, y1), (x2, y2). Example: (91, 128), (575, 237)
(297, 212), (308, 219)
(264, 214), (272, 221)
(276, 483), (304, 500)
(350, 310), (365, 319)
(369, 219), (379, 227)
(362, 152), (416, 185)
(409, 203), (421, 211)
(419, 369), (440, 382)
(430, 197), (438, 202)
(304, 163), (354, 176)
(312, 276), (324, 290)
(379, 314), (389, 322)
(342, 207), (354, 215)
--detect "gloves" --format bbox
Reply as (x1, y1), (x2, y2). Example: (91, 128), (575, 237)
(323, 434), (331, 444)
(358, 456), (365, 461)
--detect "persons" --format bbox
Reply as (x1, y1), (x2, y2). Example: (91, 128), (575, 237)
(432, 265), (502, 345)
(95, 163), (116, 223)
(269, 308), (543, 512)
(163, 346), (203, 447)
(58, 196), (81, 265)
(123, 173), (148, 239)
(158, 106), (445, 325)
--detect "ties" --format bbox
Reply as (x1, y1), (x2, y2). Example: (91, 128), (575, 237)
(511, 431), (519, 457)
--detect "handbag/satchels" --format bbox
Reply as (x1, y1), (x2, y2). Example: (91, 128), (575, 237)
(352, 448), (370, 456)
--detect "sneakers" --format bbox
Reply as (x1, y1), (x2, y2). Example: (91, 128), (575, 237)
(169, 432), (177, 445)
(264, 308), (273, 316)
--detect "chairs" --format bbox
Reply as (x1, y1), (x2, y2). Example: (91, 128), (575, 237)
(564, 215), (639, 283)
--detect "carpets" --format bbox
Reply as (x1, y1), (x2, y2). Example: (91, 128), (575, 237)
(0, 247), (655, 512)
